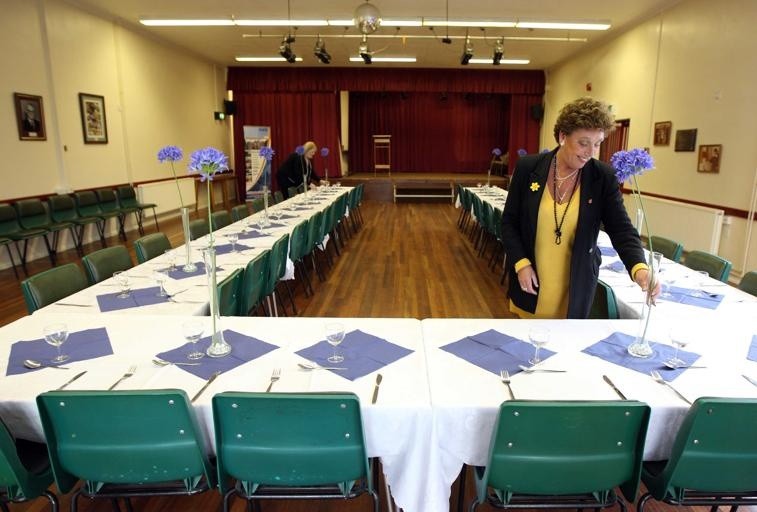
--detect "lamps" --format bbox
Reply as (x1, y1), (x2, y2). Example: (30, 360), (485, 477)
(277, 0), (508, 69)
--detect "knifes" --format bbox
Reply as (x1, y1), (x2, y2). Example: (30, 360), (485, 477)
(56, 370), (87, 391)
(740, 374), (756, 387)
(602, 374), (627, 400)
(190, 370), (221, 403)
(55, 302), (92, 307)
(370, 373), (382, 404)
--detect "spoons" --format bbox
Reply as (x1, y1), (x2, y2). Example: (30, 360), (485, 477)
(517, 364), (567, 373)
(152, 358), (199, 367)
(24, 359), (70, 370)
(661, 360), (707, 368)
(296, 362), (347, 371)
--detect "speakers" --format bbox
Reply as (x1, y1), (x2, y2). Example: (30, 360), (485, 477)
(533, 106), (540, 118)
(226, 101), (235, 115)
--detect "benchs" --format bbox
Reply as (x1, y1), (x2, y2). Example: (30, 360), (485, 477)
(392, 176), (456, 204)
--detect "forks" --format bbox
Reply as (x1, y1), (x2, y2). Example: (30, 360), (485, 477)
(499, 367), (514, 399)
(649, 370), (693, 406)
(264, 367), (281, 392)
(109, 364), (137, 390)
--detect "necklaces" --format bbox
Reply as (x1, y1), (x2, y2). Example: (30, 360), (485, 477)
(552, 154), (582, 245)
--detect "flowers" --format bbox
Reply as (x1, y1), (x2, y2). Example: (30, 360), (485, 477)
(257, 144), (275, 185)
(602, 144), (641, 220)
(295, 145), (330, 175)
(181, 147), (230, 250)
(615, 146), (658, 347)
(156, 144), (190, 208)
(488, 142), (551, 176)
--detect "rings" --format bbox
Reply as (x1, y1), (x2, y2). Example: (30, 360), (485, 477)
(522, 287), (527, 291)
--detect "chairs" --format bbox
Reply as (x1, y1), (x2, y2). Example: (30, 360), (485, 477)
(474, 398), (651, 512)
(36, 389), (223, 512)
(1, 424), (60, 512)
(213, 391), (381, 512)
(637, 397), (757, 512)
(460, 176), (757, 318)
(0, 176), (363, 316)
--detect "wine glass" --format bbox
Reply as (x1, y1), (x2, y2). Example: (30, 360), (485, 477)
(664, 325), (689, 367)
(42, 318), (70, 363)
(182, 321), (206, 360)
(112, 183), (340, 299)
(476, 183), (506, 202)
(646, 250), (709, 298)
(527, 322), (550, 365)
(323, 322), (345, 364)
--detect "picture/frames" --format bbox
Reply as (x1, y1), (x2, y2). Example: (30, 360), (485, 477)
(10, 88), (47, 142)
(696, 141), (724, 174)
(77, 90), (111, 146)
(652, 117), (672, 147)
(674, 127), (700, 151)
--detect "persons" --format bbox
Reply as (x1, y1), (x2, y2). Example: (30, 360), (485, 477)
(276, 141), (330, 201)
(501, 95), (662, 319)
(22, 103), (40, 136)
